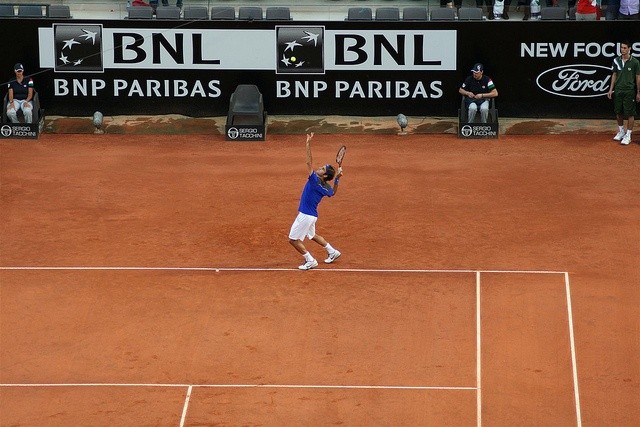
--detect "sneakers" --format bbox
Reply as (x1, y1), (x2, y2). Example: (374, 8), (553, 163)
(620, 135), (632, 144)
(324, 250), (341, 263)
(613, 132), (625, 140)
(298, 258), (318, 270)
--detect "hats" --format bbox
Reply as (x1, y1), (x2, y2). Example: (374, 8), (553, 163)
(471, 63), (483, 72)
(14, 62), (24, 72)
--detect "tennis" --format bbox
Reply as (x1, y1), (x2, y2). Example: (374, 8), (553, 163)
(291, 57), (296, 62)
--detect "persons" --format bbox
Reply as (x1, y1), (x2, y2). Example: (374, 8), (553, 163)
(575, 0), (597, 20)
(439, 1), (463, 19)
(608, 41), (639, 145)
(6, 63), (33, 125)
(288, 132), (342, 271)
(618, 0), (618, 19)
(459, 63), (499, 123)
(515, 0), (533, 20)
(485, 0), (512, 19)
(161, 0), (183, 17)
(618, 0), (640, 20)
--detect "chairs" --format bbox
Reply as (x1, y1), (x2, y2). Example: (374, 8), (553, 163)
(155, 6), (181, 20)
(457, 8), (485, 20)
(237, 6), (263, 20)
(128, 6), (154, 19)
(541, 6), (565, 20)
(0, 6), (15, 18)
(0, 88), (46, 140)
(375, 7), (400, 21)
(429, 8), (455, 20)
(265, 6), (291, 21)
(347, 7), (373, 20)
(48, 4), (71, 18)
(459, 93), (499, 139)
(402, 7), (427, 20)
(225, 83), (267, 142)
(569, 9), (576, 19)
(17, 5), (44, 18)
(183, 6), (209, 20)
(210, 6), (235, 21)
